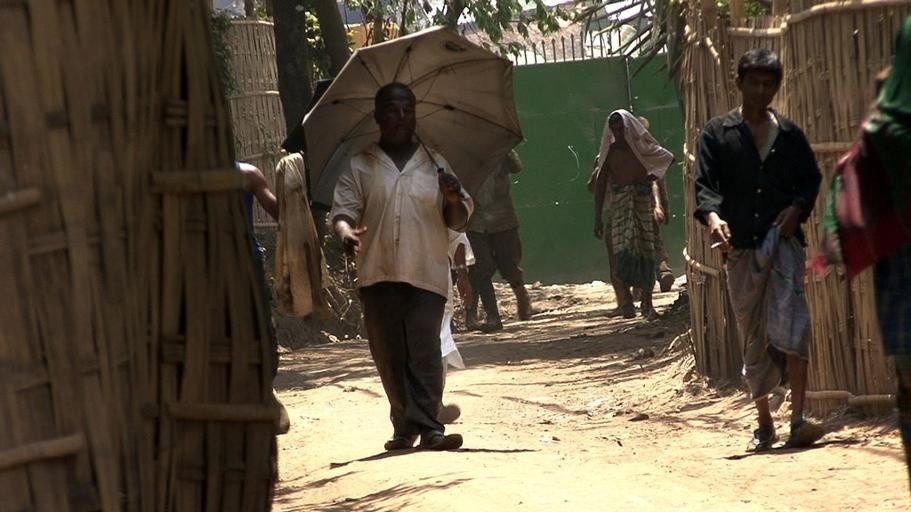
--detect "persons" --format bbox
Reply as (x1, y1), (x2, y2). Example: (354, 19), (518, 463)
(810, 17), (911, 495)
(450, 263), (482, 334)
(236, 161), (290, 435)
(632, 116), (675, 299)
(465, 149), (532, 332)
(436, 227), (476, 424)
(593, 109), (676, 322)
(691, 50), (824, 452)
(329, 82), (475, 451)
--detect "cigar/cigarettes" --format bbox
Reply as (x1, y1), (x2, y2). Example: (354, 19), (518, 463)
(711, 238), (729, 249)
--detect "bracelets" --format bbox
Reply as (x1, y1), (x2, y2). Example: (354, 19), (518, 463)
(456, 264), (469, 275)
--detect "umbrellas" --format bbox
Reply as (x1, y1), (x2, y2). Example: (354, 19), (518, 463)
(302, 25), (526, 207)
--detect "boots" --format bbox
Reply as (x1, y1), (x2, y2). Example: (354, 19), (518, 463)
(513, 283), (532, 319)
(465, 294), (502, 331)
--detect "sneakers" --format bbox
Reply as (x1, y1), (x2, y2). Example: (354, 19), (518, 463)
(787, 420), (822, 445)
(385, 434), (413, 449)
(747, 422), (778, 452)
(660, 273), (675, 292)
(641, 302), (659, 318)
(621, 303), (636, 318)
(420, 430), (462, 450)
